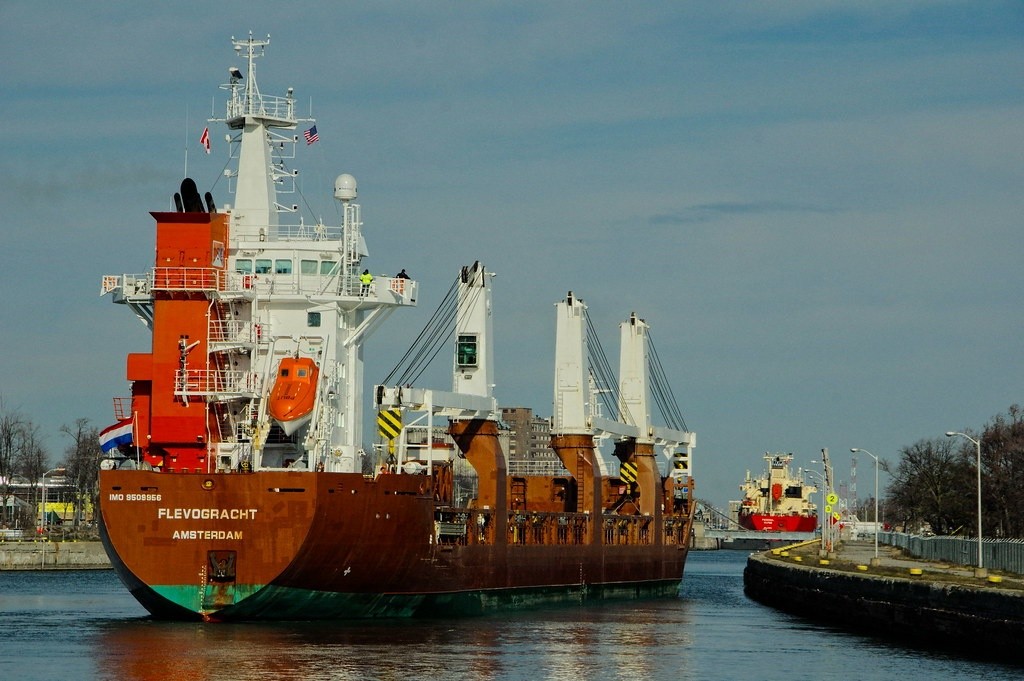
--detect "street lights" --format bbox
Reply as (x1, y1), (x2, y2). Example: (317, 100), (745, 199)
(850, 447), (880, 558)
(944, 431), (983, 569)
(803, 458), (837, 553)
(41, 468), (65, 570)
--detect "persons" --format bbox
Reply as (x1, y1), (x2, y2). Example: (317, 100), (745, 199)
(379, 467), (391, 476)
(395, 269), (411, 279)
(359, 269), (373, 297)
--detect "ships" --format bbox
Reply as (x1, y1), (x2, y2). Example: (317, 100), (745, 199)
(90, 29), (698, 621)
(737, 450), (817, 533)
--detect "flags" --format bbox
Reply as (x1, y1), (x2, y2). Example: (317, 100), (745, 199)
(200, 127), (210, 154)
(98, 416), (135, 454)
(303, 125), (319, 145)
